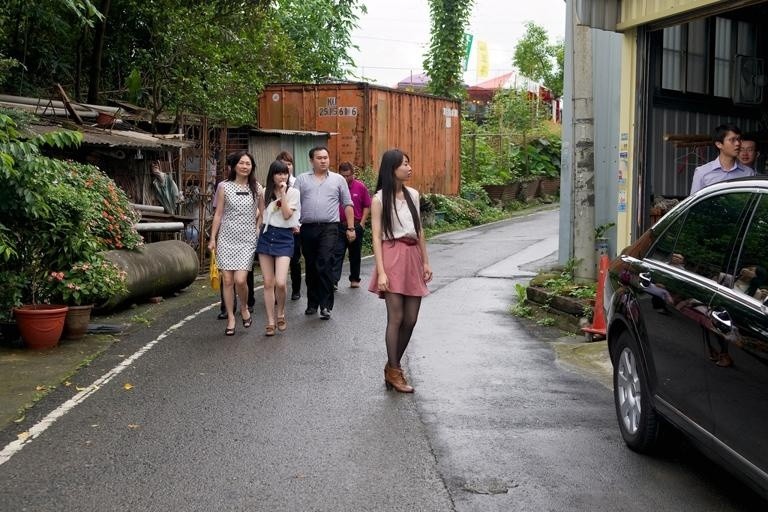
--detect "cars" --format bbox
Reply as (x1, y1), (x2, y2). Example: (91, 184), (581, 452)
(603, 174), (766, 512)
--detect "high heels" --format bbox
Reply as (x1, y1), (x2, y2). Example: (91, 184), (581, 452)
(384, 364), (415, 393)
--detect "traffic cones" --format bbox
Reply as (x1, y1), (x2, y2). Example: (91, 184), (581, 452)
(580, 252), (609, 336)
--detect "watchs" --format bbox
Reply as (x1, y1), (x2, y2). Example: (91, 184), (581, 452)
(346, 227), (355, 231)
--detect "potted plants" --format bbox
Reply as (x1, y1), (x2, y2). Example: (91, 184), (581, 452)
(11, 234), (69, 351)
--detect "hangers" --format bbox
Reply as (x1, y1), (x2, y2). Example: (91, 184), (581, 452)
(674, 139), (709, 166)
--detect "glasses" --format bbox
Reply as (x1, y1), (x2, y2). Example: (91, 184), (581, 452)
(724, 137), (742, 143)
(740, 149), (755, 153)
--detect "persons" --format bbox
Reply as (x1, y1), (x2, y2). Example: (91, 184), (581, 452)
(213, 153), (255, 319)
(333, 160), (373, 290)
(297, 146), (357, 320)
(274, 152), (302, 306)
(255, 160), (299, 336)
(207, 151), (265, 336)
(607, 251), (768, 367)
(689, 123), (757, 195)
(735, 132), (761, 174)
(368, 149), (433, 393)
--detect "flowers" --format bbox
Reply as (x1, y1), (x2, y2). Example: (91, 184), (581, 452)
(50, 256), (144, 306)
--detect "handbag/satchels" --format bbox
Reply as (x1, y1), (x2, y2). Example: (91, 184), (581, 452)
(210, 263), (221, 293)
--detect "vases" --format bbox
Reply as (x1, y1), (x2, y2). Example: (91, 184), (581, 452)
(63, 302), (94, 344)
(96, 109), (113, 128)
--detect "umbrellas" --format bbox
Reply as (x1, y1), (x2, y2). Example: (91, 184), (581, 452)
(152, 159), (178, 215)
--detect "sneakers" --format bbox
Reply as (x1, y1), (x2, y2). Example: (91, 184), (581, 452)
(218, 280), (359, 336)
